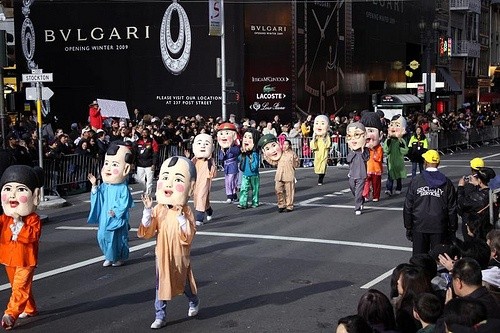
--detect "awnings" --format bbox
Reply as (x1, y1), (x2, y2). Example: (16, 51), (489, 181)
(435, 69), (462, 96)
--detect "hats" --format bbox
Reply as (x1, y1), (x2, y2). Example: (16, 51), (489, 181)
(422, 149), (440, 163)
(89, 101), (98, 107)
(470, 157), (487, 170)
(476, 167), (496, 179)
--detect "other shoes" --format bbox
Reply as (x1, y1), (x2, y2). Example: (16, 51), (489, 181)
(356, 211), (361, 215)
(19, 312), (29, 319)
(278, 207), (294, 213)
(227, 199), (232, 204)
(196, 221), (203, 226)
(373, 198), (378, 201)
(396, 191), (401, 194)
(103, 260), (111, 267)
(385, 190), (391, 195)
(207, 209), (213, 220)
(2, 314), (16, 331)
(112, 260), (124, 266)
(237, 205), (246, 208)
(318, 183), (322, 185)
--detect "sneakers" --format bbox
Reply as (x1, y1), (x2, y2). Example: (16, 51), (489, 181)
(150, 319), (166, 328)
(188, 295), (200, 317)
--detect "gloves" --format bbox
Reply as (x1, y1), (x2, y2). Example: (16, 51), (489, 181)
(448, 222), (458, 232)
(406, 229), (413, 242)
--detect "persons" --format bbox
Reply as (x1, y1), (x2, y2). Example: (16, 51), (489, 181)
(184, 134), (219, 226)
(310, 114), (331, 185)
(259, 134), (300, 212)
(86, 144), (136, 266)
(403, 149), (458, 254)
(383, 114), (409, 195)
(0, 100), (500, 190)
(216, 123), (239, 203)
(0, 166), (41, 330)
(360, 110), (384, 202)
(346, 122), (369, 215)
(138, 155), (201, 328)
(237, 128), (260, 208)
(336, 158), (500, 333)
(407, 127), (427, 179)
